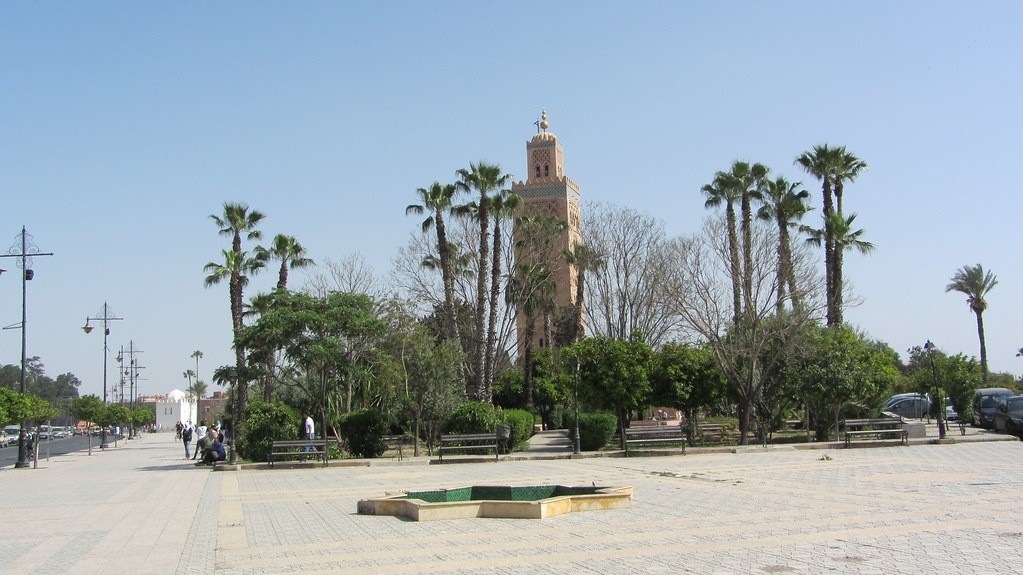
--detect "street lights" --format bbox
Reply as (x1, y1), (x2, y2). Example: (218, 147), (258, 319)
(228, 368), (239, 464)
(83, 317), (109, 448)
(116, 351), (134, 439)
(111, 386), (118, 404)
(572, 354), (593, 456)
(924, 337), (948, 439)
(125, 367), (138, 404)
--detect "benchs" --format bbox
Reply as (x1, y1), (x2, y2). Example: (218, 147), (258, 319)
(696, 423), (728, 435)
(776, 419), (807, 432)
(382, 435), (408, 461)
(844, 416), (908, 449)
(624, 425), (687, 457)
(271, 439), (328, 468)
(438, 432), (498, 463)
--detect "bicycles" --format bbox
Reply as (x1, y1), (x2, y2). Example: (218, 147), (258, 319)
(175, 433), (183, 441)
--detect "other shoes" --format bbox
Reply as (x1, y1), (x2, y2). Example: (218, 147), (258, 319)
(194, 461), (206, 467)
(190, 457), (196, 460)
(182, 457), (191, 460)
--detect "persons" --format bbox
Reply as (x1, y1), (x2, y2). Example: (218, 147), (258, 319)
(26, 435), (34, 460)
(302, 410), (322, 462)
(659, 409), (667, 420)
(175, 415), (228, 466)
(143, 423), (156, 433)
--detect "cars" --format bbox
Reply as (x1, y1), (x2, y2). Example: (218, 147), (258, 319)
(944, 395), (960, 421)
(880, 393), (931, 403)
(77, 425), (103, 436)
(53, 427), (65, 438)
(0, 431), (9, 448)
(104, 428), (111, 435)
(64, 425), (76, 436)
(886, 397), (932, 410)
(887, 399), (935, 420)
(23, 426), (38, 442)
(992, 396), (1023, 441)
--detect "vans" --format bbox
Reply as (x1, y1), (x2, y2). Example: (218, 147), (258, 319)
(40, 425), (53, 439)
(971, 387), (1015, 429)
(4, 424), (22, 446)
